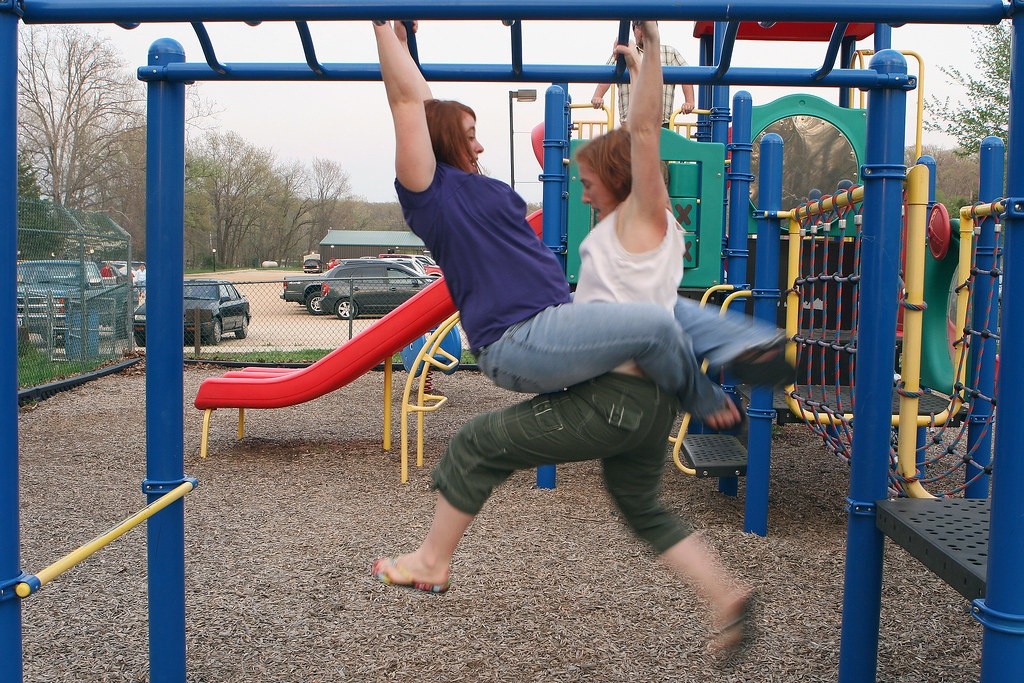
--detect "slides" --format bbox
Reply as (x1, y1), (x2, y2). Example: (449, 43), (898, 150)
(193, 204), (542, 410)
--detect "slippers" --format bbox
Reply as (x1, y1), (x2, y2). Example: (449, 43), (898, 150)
(370, 556), (451, 593)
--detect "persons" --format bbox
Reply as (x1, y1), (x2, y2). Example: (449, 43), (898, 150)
(373, 21), (759, 670)
(591, 21), (695, 129)
(373, 13), (784, 425)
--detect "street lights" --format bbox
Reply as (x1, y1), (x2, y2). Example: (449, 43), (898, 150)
(213, 248), (216, 272)
(91, 248), (94, 260)
(331, 245), (334, 258)
(508, 88), (536, 190)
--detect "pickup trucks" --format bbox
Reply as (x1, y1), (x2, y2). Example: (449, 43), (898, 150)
(279, 258), (336, 314)
(17, 260), (140, 350)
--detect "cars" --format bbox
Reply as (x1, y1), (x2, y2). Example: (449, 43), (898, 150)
(328, 253), (443, 280)
(318, 265), (431, 320)
(69, 261), (146, 285)
(133, 278), (251, 345)
(303, 258), (323, 272)
